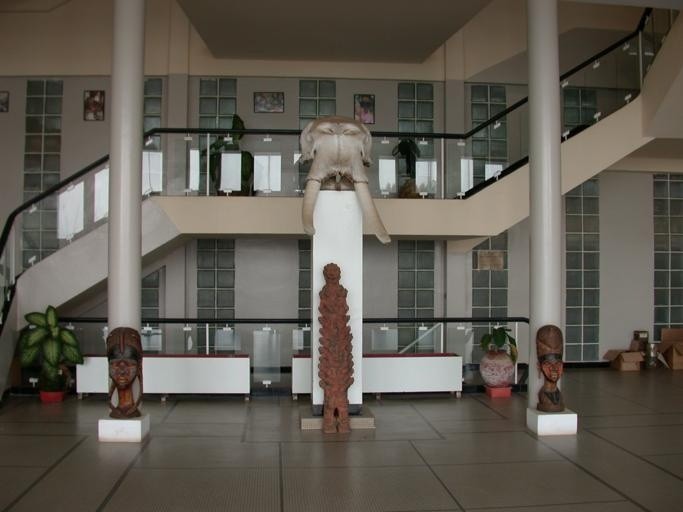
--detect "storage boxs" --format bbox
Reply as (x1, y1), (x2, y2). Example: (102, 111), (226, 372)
(602, 328), (683, 372)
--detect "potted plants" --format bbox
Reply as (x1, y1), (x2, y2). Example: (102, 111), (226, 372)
(477, 323), (519, 389)
(17, 304), (84, 404)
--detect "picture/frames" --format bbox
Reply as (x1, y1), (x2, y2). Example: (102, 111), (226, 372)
(83, 90), (105, 121)
(354, 94), (375, 125)
(253, 92), (285, 113)
